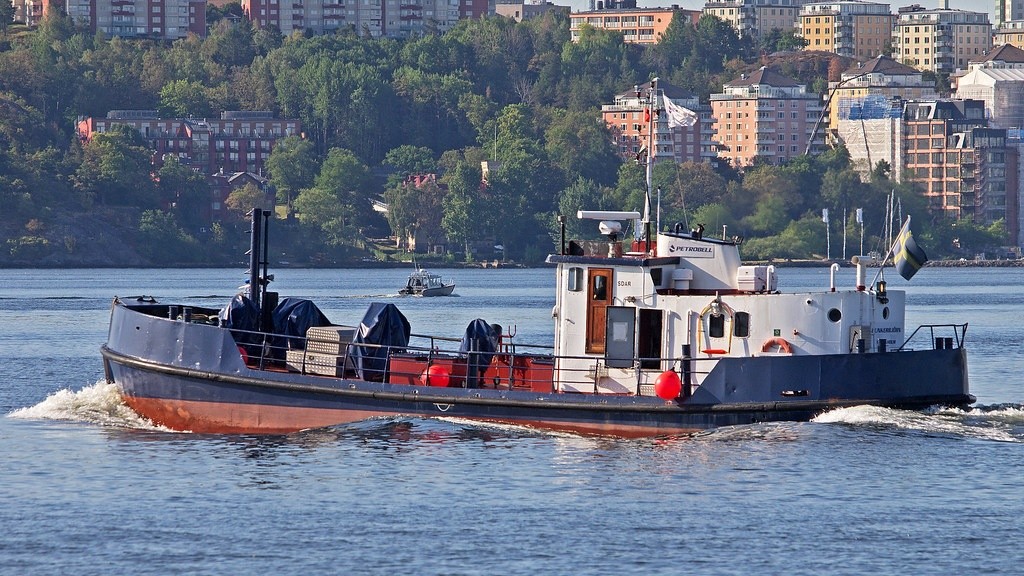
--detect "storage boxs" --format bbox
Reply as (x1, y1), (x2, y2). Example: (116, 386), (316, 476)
(285, 326), (357, 376)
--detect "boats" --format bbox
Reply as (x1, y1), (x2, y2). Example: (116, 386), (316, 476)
(100, 78), (977, 443)
(397, 254), (456, 297)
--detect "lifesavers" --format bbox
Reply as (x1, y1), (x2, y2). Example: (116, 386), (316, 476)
(760, 337), (793, 353)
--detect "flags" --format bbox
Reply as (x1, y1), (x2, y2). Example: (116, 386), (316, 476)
(894, 220), (928, 281)
(662, 94), (698, 130)
